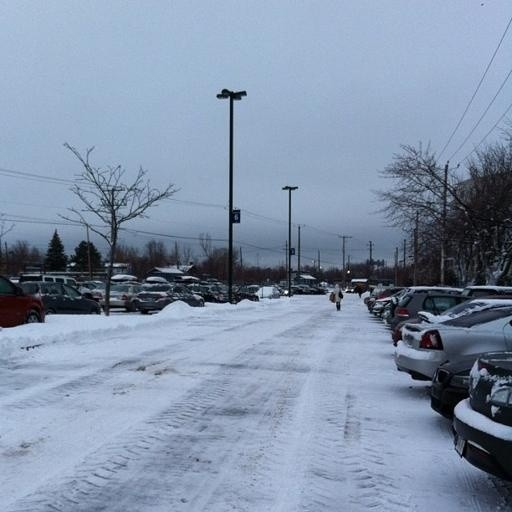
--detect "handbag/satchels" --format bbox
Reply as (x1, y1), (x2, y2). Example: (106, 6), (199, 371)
(339, 293), (343, 298)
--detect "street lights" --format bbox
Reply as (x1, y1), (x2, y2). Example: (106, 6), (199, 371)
(282, 185), (297, 295)
(217, 90), (246, 300)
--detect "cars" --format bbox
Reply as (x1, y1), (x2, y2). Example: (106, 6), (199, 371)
(430, 350), (512, 481)
(394, 299), (512, 381)
(291, 284), (326, 294)
(20, 280), (101, 315)
(0, 275), (45, 326)
(366, 287), (511, 332)
(241, 284), (282, 301)
(77, 278), (243, 315)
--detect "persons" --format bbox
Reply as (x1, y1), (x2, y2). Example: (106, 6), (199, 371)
(357, 283), (362, 299)
(332, 283), (343, 311)
